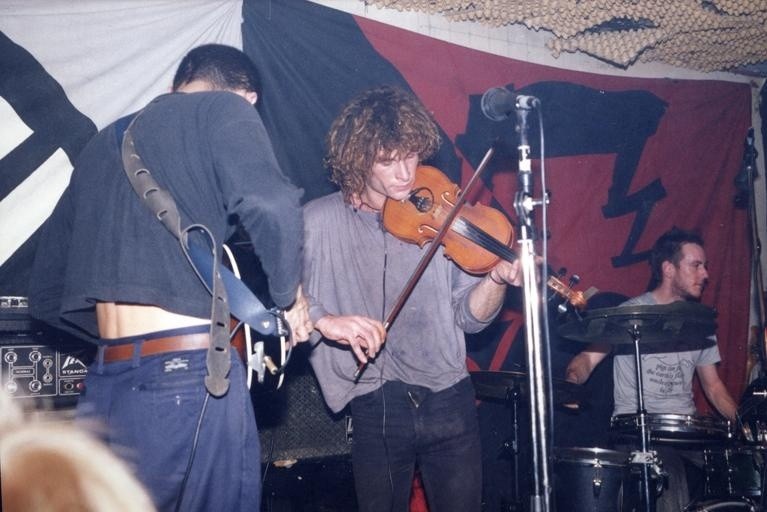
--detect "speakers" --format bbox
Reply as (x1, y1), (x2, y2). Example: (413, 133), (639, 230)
(244, 343), (354, 471)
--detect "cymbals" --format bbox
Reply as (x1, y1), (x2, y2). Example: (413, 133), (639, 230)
(556, 303), (717, 345)
(470, 371), (583, 405)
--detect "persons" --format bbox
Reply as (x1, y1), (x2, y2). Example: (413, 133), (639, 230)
(28, 42), (313, 512)
(0, 418), (157, 512)
(302, 83), (544, 512)
(560, 227), (753, 512)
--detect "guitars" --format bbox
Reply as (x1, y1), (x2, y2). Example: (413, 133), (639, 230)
(222, 241), (286, 395)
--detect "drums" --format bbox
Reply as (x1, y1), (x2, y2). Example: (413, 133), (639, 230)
(703, 443), (767, 498)
(547, 447), (628, 512)
(610, 413), (733, 448)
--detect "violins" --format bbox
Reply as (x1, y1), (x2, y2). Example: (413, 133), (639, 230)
(381, 164), (599, 313)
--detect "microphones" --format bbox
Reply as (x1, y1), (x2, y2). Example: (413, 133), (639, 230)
(748, 127), (759, 178)
(480, 86), (540, 121)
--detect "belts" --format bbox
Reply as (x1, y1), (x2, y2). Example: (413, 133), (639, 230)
(104, 333), (210, 362)
